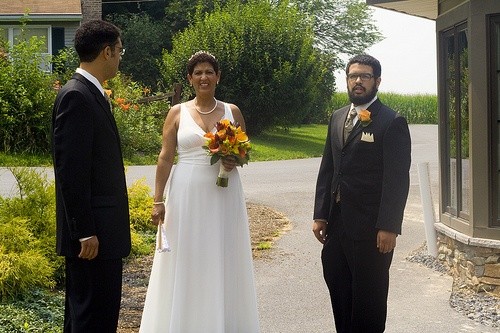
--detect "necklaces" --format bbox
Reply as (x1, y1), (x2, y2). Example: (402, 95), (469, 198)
(194, 96), (218, 114)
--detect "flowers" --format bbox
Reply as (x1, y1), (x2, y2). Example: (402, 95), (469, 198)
(202, 119), (252, 188)
(356, 109), (372, 129)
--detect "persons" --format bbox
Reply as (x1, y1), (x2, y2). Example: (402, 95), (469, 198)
(139, 50), (260, 333)
(312, 55), (411, 333)
(52, 20), (131, 333)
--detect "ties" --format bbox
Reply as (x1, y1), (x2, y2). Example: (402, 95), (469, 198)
(104, 91), (113, 114)
(343, 109), (358, 144)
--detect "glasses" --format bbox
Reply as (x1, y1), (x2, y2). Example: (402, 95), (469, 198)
(112, 44), (127, 56)
(347, 72), (374, 81)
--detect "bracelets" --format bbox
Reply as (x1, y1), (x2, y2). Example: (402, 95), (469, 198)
(153, 202), (164, 205)
